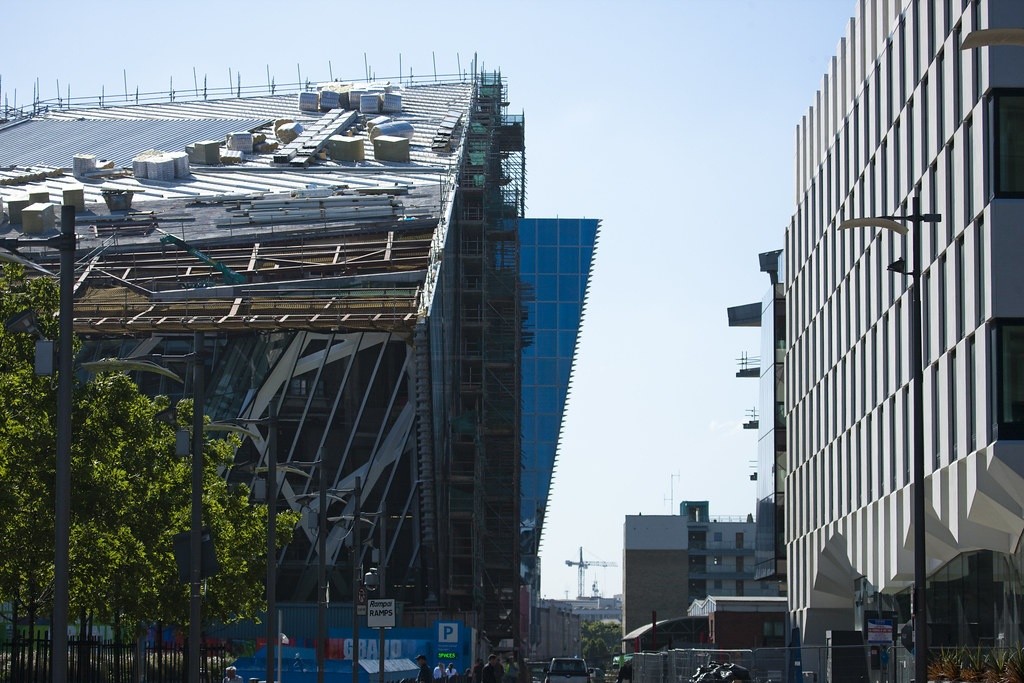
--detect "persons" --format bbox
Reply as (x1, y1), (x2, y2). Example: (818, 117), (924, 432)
(415, 654), (522, 683)
(593, 659), (634, 683)
(223, 666), (244, 683)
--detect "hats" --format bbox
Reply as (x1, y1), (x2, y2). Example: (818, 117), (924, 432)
(414, 654), (427, 661)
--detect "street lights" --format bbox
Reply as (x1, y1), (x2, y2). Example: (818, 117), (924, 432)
(277, 446), (326, 683)
(340, 501), (386, 683)
(118, 328), (263, 683)
(209, 399), (276, 678)
(327, 475), (361, 681)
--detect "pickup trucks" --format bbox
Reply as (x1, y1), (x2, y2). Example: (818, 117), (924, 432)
(542, 657), (595, 683)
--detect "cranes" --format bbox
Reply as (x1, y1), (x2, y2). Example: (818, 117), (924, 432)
(565, 546), (621, 598)
